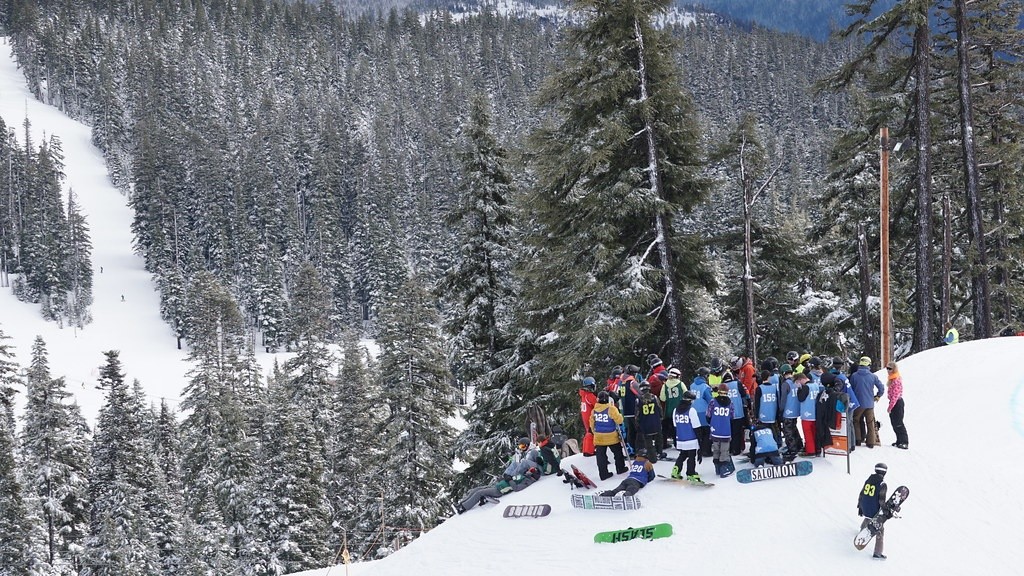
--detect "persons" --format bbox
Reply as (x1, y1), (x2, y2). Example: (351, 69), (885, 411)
(452, 426), (567, 516)
(101, 267), (103, 273)
(857, 462), (892, 560)
(886, 362), (909, 449)
(940, 322), (959, 346)
(598, 448), (656, 497)
(578, 350), (885, 482)
(121, 294), (125, 302)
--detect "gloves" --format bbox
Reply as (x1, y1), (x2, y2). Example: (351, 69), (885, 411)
(503, 474), (512, 482)
(859, 508), (863, 516)
(886, 511), (892, 519)
(557, 470), (563, 476)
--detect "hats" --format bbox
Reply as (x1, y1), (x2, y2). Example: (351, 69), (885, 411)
(875, 463), (888, 472)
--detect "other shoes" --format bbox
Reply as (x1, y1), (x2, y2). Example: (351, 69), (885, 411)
(687, 474), (700, 482)
(585, 440), (679, 481)
(451, 502), (465, 514)
(700, 437), (836, 477)
(847, 433), (908, 452)
(873, 553), (887, 559)
(672, 467), (683, 480)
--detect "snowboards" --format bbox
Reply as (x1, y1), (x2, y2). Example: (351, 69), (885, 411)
(854, 485), (908, 551)
(594, 524), (672, 542)
(570, 464), (597, 487)
(570, 493), (641, 511)
(737, 460), (813, 483)
(657, 474), (714, 487)
(562, 468), (589, 490)
(625, 457), (676, 461)
(503, 504), (551, 517)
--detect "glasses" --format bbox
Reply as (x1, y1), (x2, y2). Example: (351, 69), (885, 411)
(519, 444), (525, 448)
(885, 366), (893, 371)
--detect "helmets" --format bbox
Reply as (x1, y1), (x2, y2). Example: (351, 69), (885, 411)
(611, 354), (662, 381)
(597, 391), (608, 404)
(583, 377), (596, 386)
(682, 391), (696, 401)
(638, 381), (651, 390)
(517, 436), (531, 447)
(529, 467), (540, 481)
(717, 384), (729, 394)
(695, 351), (872, 382)
(669, 368), (682, 377)
